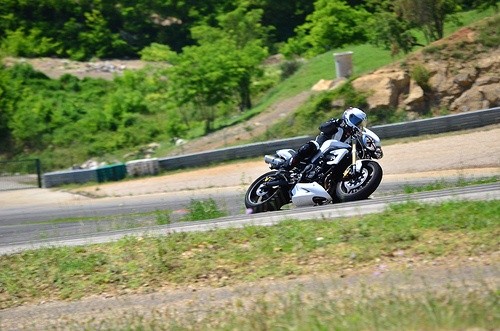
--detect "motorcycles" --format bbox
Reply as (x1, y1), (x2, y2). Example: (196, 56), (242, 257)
(245, 128), (382, 214)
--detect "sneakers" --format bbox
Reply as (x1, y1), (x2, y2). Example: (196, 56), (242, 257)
(277, 164), (292, 180)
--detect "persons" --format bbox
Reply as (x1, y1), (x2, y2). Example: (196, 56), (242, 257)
(275, 108), (367, 185)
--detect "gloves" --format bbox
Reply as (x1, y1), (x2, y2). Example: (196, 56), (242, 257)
(344, 125), (359, 136)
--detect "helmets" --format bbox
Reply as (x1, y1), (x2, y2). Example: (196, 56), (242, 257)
(342, 108), (366, 129)
